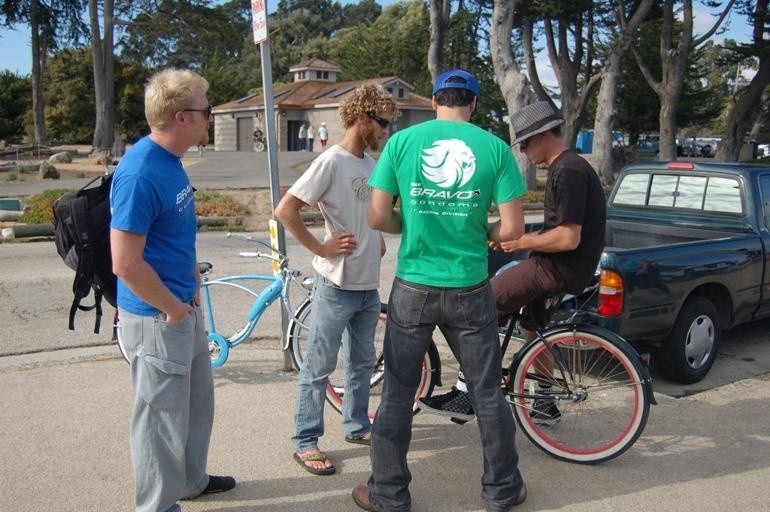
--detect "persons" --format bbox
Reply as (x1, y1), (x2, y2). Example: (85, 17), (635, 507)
(418, 100), (603, 423)
(298, 123), (307, 153)
(318, 122), (328, 151)
(350, 70), (526, 511)
(708, 140), (719, 157)
(273, 80), (401, 475)
(688, 134), (703, 157)
(108, 67), (237, 511)
(306, 126), (316, 153)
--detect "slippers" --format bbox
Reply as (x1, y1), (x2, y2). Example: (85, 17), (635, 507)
(345, 432), (371, 445)
(293, 453), (335, 475)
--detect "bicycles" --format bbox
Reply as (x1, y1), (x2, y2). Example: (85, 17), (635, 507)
(325, 279), (657, 466)
(115, 232), (386, 396)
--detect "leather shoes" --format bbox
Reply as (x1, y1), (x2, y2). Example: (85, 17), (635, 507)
(352, 486), (373, 511)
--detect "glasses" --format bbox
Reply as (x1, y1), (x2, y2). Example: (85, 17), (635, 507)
(365, 111), (389, 128)
(184, 105), (210, 120)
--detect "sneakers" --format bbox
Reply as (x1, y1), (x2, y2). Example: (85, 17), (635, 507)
(416, 386), (475, 420)
(528, 401), (561, 425)
(203, 475), (235, 493)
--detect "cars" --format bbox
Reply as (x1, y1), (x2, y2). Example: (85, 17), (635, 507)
(610, 130), (770, 159)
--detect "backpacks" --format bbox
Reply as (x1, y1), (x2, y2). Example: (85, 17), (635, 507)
(53, 172), (116, 333)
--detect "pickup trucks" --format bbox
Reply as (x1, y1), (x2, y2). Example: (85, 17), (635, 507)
(484, 161), (770, 384)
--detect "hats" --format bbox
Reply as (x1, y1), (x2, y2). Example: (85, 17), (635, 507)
(509, 101), (564, 148)
(432, 69), (480, 95)
